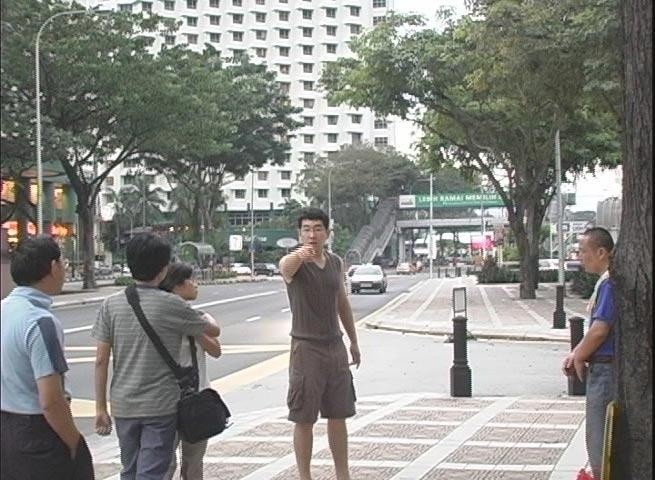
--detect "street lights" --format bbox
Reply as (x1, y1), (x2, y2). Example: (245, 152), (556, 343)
(34, 5), (113, 237)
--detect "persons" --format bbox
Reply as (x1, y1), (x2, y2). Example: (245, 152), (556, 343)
(90, 232), (220, 480)
(1, 235), (93, 480)
(159, 262), (221, 480)
(561, 226), (618, 480)
(278, 207), (361, 480)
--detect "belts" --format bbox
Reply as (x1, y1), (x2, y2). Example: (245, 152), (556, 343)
(586, 355), (615, 363)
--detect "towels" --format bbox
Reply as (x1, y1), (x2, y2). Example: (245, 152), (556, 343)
(586, 270), (611, 315)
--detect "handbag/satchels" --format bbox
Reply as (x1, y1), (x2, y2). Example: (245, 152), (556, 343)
(177, 389), (232, 443)
(179, 366), (199, 397)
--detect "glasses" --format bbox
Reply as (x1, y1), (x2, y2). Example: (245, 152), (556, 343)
(59, 259), (69, 268)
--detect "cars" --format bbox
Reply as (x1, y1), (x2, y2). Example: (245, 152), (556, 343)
(252, 262), (283, 277)
(395, 262), (416, 276)
(492, 257), (582, 274)
(78, 260), (130, 276)
(222, 262), (252, 276)
(346, 263), (388, 294)
